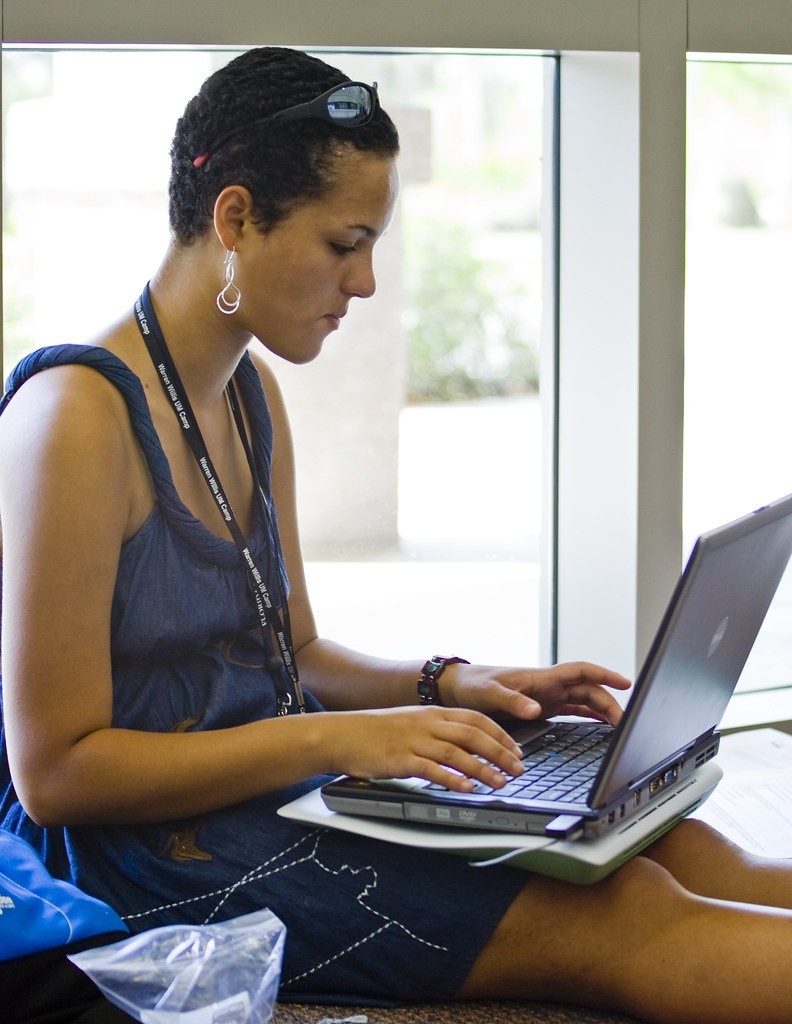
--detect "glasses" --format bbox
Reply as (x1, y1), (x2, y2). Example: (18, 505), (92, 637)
(192, 80), (380, 169)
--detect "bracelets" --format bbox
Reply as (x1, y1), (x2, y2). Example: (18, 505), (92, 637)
(417, 655), (469, 707)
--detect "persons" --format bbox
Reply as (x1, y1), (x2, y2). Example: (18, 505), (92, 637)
(0, 46), (792, 1024)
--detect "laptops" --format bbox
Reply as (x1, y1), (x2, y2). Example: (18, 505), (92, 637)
(320, 496), (792, 840)
